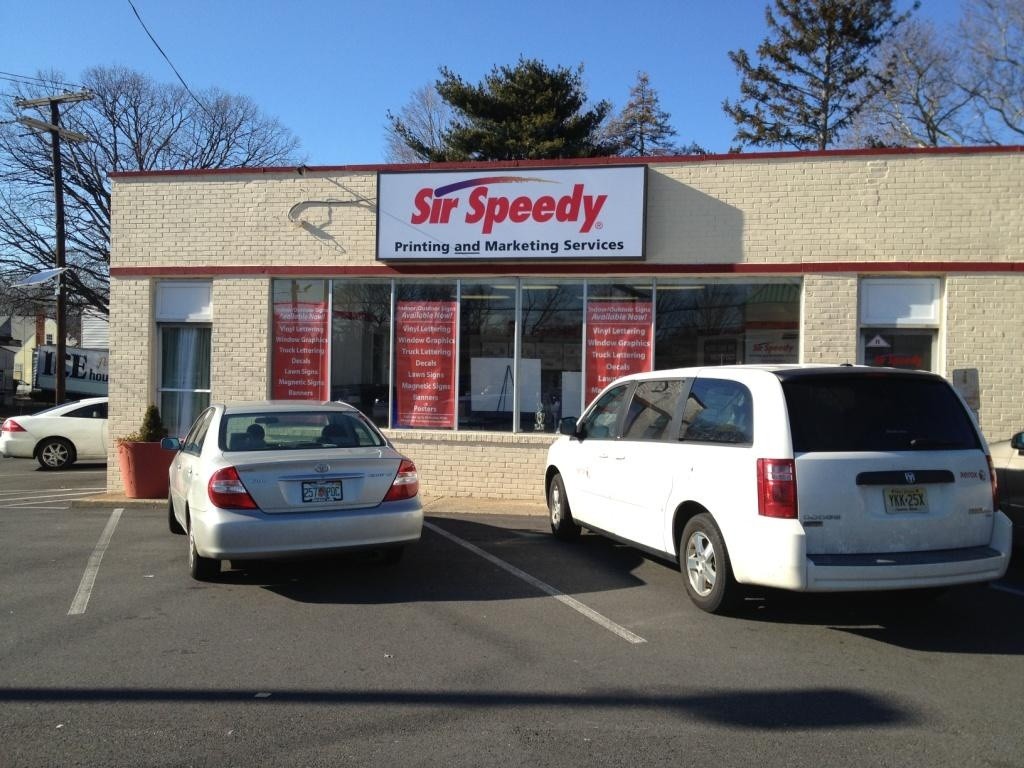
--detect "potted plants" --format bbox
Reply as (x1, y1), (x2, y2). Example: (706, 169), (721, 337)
(112, 405), (179, 498)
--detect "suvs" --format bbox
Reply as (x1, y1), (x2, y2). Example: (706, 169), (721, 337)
(544, 362), (1009, 614)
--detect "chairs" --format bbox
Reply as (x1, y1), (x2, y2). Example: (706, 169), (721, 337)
(230, 432), (247, 449)
(321, 425), (358, 445)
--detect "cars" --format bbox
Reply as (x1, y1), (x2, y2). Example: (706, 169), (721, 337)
(0, 396), (109, 471)
(158, 397), (423, 580)
